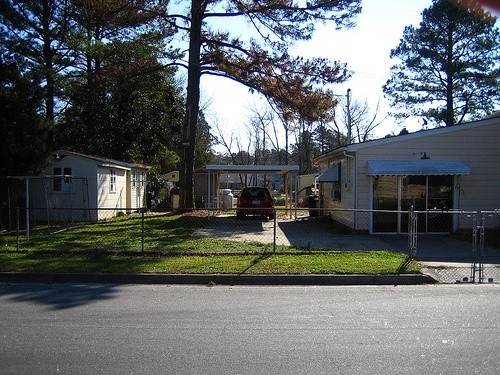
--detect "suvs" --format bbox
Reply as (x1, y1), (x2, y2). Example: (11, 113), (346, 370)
(234, 186), (275, 219)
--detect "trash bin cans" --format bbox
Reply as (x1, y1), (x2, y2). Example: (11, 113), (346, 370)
(307, 195), (320, 217)
(223, 189), (233, 209)
